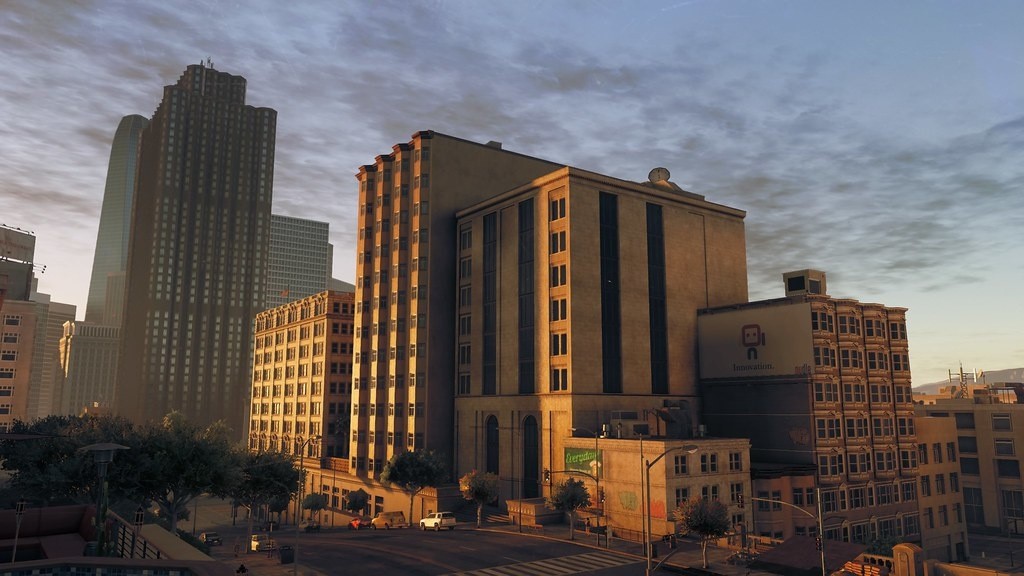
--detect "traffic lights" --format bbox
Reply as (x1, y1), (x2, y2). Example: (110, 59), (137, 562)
(738, 494), (744, 508)
(816, 538), (821, 551)
(600, 491), (606, 503)
(544, 470), (549, 481)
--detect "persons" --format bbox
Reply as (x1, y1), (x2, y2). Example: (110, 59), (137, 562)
(233, 536), (240, 557)
(585, 518), (591, 536)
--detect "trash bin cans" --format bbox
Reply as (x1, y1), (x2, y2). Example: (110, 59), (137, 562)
(650, 544), (658, 558)
(278, 544), (294, 565)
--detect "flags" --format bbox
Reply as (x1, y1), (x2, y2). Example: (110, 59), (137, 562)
(280, 290), (289, 296)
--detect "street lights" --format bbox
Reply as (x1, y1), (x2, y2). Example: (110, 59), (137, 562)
(496, 427), (521, 533)
(572, 427), (611, 547)
(80, 443), (131, 557)
(646, 444), (698, 576)
(193, 493), (209, 536)
(294, 435), (321, 576)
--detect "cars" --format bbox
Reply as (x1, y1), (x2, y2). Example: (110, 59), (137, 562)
(348, 517), (370, 531)
(299, 521), (320, 530)
(251, 535), (277, 552)
(198, 532), (223, 546)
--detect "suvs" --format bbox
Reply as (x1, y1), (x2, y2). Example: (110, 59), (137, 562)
(420, 512), (456, 532)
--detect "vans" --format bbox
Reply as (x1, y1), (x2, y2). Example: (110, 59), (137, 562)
(371, 511), (408, 530)
